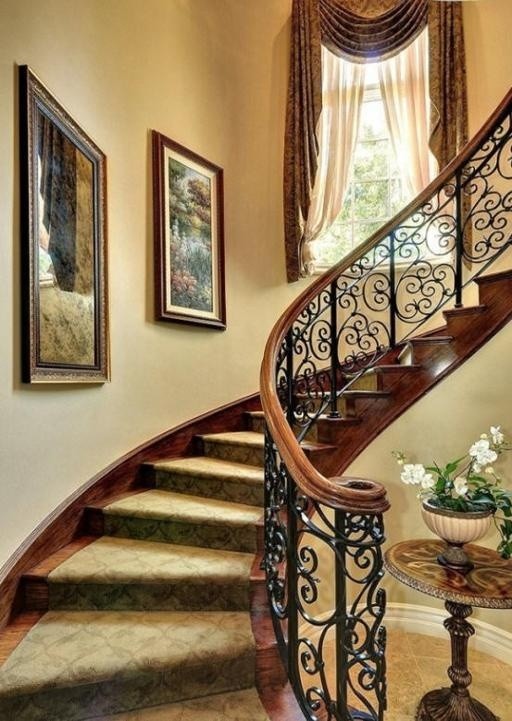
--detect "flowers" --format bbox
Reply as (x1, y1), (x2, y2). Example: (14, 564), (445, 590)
(388, 422), (512, 565)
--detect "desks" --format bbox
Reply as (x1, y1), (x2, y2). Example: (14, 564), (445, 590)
(382, 540), (512, 721)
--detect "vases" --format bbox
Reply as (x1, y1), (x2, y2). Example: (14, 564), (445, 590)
(418, 497), (497, 575)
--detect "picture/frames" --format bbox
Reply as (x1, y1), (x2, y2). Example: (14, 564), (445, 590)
(17, 61), (115, 392)
(147, 128), (229, 332)
